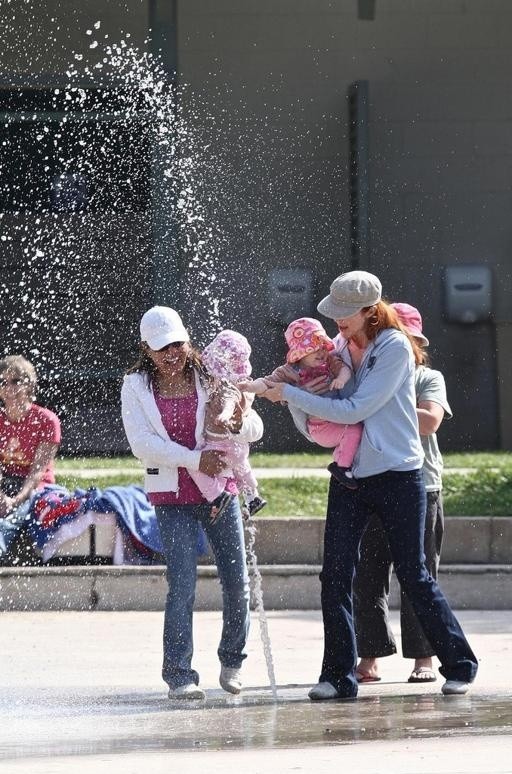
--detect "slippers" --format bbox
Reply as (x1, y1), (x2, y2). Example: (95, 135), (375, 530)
(408, 669), (437, 682)
(355, 666), (381, 682)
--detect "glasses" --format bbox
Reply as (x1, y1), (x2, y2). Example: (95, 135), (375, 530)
(1, 378), (30, 388)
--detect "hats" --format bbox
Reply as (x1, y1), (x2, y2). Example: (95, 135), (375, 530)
(389, 302), (429, 348)
(284, 317), (336, 365)
(200, 329), (253, 380)
(317, 270), (383, 320)
(139, 305), (190, 351)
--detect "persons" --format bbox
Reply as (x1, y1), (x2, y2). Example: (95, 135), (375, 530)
(186, 329), (267, 526)
(257, 269), (478, 700)
(238, 317), (364, 491)
(353, 302), (454, 684)
(0, 354), (62, 558)
(122, 305), (264, 702)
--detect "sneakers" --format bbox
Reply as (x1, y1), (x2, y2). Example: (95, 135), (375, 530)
(308, 682), (341, 700)
(208, 490), (236, 527)
(442, 680), (473, 694)
(168, 682), (206, 699)
(220, 662), (242, 694)
(327, 461), (360, 490)
(240, 496), (267, 521)
